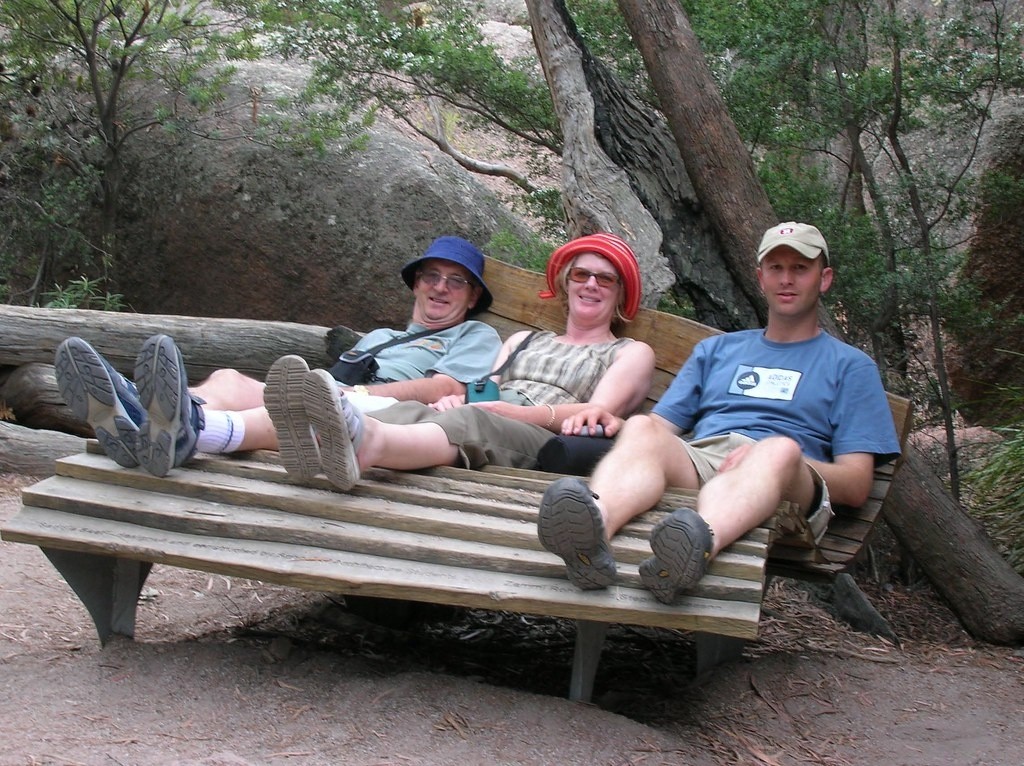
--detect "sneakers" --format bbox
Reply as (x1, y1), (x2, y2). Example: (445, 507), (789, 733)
(54, 336), (149, 468)
(133, 333), (208, 477)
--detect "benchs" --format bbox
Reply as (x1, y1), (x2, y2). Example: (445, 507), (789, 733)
(1, 253), (916, 704)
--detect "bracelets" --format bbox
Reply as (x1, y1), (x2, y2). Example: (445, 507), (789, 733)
(544, 404), (555, 429)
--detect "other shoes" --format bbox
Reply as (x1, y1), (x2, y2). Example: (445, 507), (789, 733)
(536, 475), (617, 591)
(638, 507), (715, 605)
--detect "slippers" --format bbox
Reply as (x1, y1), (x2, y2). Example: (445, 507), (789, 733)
(301, 368), (365, 491)
(262, 354), (322, 479)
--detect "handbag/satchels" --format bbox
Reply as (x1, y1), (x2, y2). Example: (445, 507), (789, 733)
(326, 350), (380, 386)
(464, 377), (500, 404)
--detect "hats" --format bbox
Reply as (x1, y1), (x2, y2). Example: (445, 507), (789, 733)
(400, 236), (493, 315)
(546, 233), (641, 321)
(757, 221), (830, 266)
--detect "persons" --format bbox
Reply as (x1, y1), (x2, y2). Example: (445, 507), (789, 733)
(55, 236), (504, 477)
(538, 220), (900, 606)
(262, 233), (656, 494)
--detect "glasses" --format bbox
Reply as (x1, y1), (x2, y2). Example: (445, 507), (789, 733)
(568, 267), (621, 287)
(417, 270), (475, 290)
